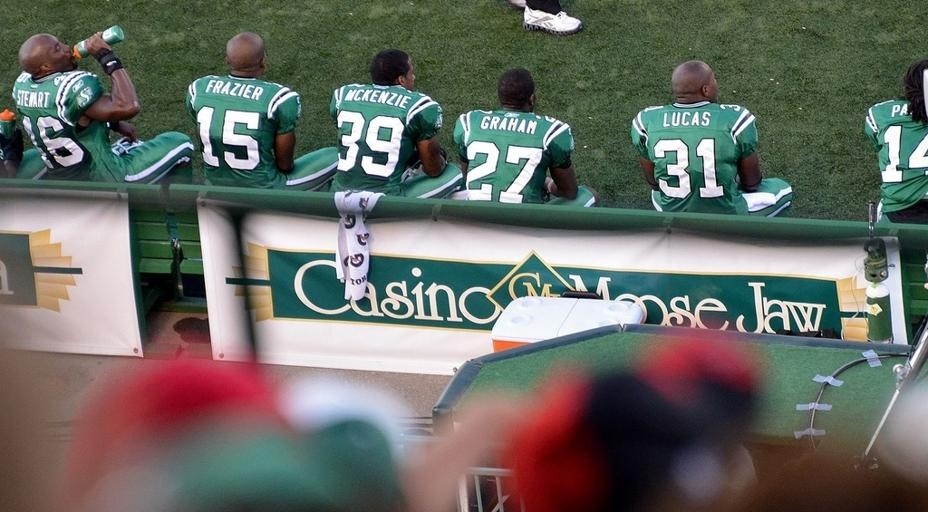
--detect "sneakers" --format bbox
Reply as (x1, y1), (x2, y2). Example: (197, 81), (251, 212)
(510, 0), (583, 35)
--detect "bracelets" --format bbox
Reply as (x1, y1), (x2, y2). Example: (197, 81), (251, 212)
(547, 179), (554, 194)
(94, 47), (123, 75)
(106, 120), (118, 131)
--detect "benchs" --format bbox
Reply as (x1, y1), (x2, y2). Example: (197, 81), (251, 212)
(129, 208), (207, 316)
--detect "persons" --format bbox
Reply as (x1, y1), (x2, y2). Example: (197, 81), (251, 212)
(329, 49), (466, 200)
(453, 67), (600, 208)
(185, 31), (339, 192)
(12, 33), (194, 185)
(632, 60), (795, 218)
(861, 58), (928, 226)
(0, 128), (49, 180)
(510, 0), (583, 36)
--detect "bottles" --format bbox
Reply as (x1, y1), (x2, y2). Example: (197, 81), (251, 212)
(0, 109), (16, 161)
(71, 25), (125, 59)
